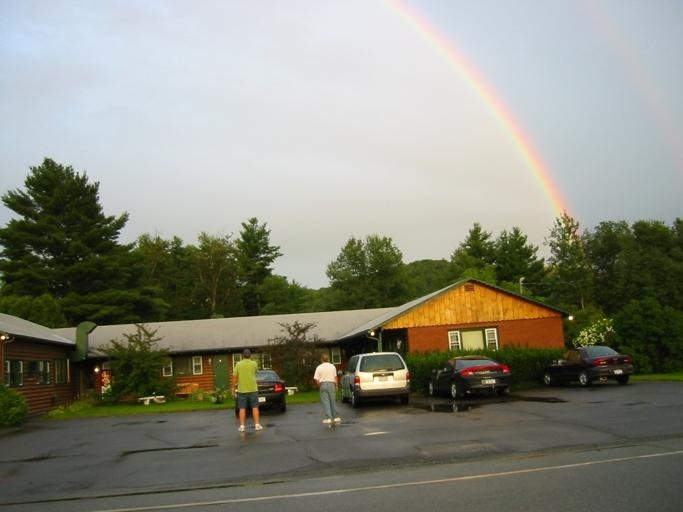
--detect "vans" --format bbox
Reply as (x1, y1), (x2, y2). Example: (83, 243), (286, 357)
(341, 352), (410, 407)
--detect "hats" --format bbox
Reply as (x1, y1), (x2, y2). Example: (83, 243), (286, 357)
(243, 349), (250, 354)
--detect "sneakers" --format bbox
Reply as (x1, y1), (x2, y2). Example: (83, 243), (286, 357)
(333, 417), (341, 422)
(238, 425), (245, 432)
(254, 424), (263, 431)
(322, 418), (332, 423)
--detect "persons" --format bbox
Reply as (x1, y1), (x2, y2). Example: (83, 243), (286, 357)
(314, 354), (343, 424)
(232, 348), (264, 433)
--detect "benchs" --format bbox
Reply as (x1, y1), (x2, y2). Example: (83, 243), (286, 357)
(173, 383), (199, 397)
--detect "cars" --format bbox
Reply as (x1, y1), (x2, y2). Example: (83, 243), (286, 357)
(543, 346), (635, 387)
(234, 369), (286, 414)
(429, 355), (511, 400)
